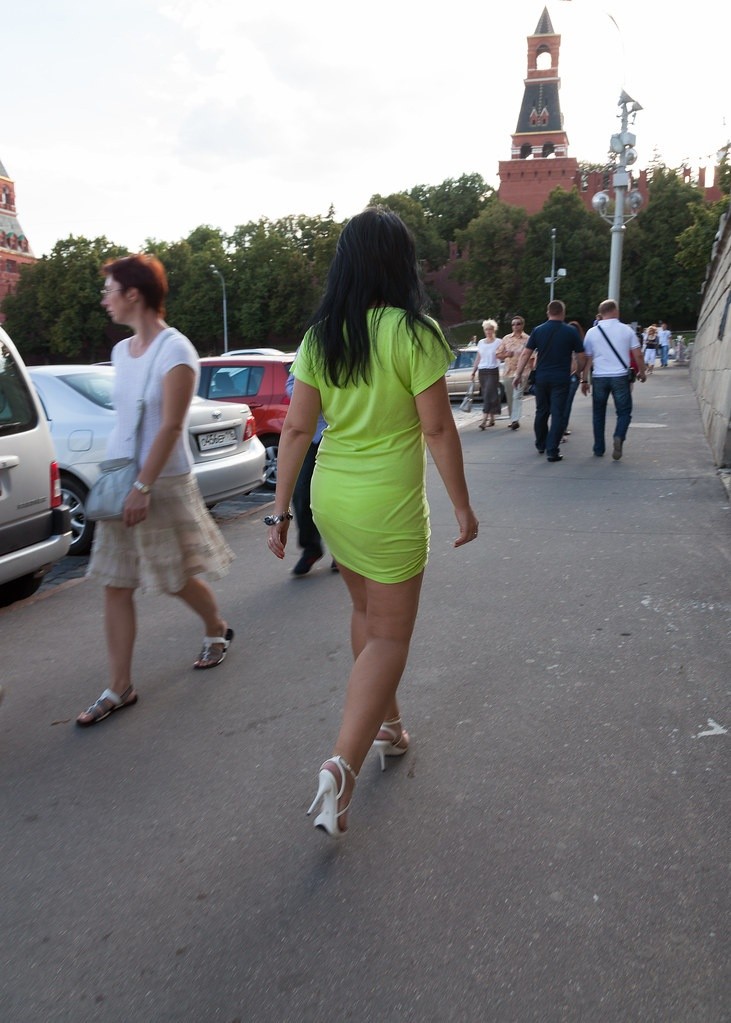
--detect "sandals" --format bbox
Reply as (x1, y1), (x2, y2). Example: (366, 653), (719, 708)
(77, 685), (138, 726)
(486, 420), (494, 427)
(194, 629), (234, 669)
(479, 423), (486, 431)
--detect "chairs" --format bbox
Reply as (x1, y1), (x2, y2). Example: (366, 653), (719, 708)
(213, 372), (238, 396)
(462, 358), (474, 367)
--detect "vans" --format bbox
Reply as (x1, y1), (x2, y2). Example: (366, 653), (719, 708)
(0, 327), (73, 597)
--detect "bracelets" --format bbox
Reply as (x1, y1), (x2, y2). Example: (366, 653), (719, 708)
(580, 380), (588, 383)
(262, 511), (294, 526)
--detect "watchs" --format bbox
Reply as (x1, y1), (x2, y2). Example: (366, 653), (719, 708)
(131, 481), (154, 495)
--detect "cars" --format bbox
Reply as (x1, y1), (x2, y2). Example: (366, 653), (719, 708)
(91, 355), (296, 491)
(210, 349), (285, 387)
(444, 347), (506, 403)
(0, 364), (267, 555)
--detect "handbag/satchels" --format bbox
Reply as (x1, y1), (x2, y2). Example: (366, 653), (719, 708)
(459, 376), (475, 413)
(85, 458), (140, 522)
(628, 367), (636, 383)
(657, 344), (662, 348)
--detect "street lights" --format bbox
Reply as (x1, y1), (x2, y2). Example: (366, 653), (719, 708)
(592, 90), (644, 302)
(544, 268), (566, 301)
(210, 265), (227, 353)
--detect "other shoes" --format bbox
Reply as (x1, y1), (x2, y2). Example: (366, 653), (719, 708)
(292, 547), (323, 574)
(539, 450), (544, 453)
(564, 431), (571, 435)
(548, 455), (563, 462)
(508, 424), (512, 427)
(560, 439), (568, 443)
(331, 561), (336, 567)
(613, 437), (622, 460)
(594, 453), (603, 457)
(512, 423), (520, 430)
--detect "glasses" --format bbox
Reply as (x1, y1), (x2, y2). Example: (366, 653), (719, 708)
(648, 333), (654, 335)
(511, 323), (522, 326)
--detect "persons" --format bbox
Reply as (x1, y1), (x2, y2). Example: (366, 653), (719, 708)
(512, 300), (586, 461)
(285, 356), (342, 579)
(265, 206), (481, 839)
(469, 318), (502, 430)
(592, 314), (672, 373)
(580, 298), (647, 461)
(74, 252), (236, 728)
(559, 313), (586, 446)
(495, 316), (538, 430)
(466, 334), (477, 365)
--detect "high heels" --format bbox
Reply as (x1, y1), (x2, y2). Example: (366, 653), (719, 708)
(374, 714), (407, 771)
(305, 756), (359, 838)
